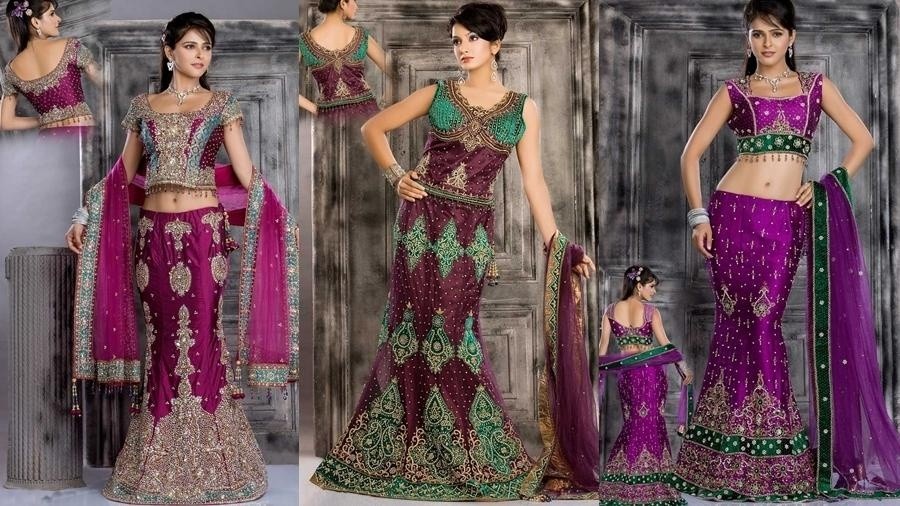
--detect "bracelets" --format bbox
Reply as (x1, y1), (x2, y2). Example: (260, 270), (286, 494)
(685, 207), (709, 229)
(383, 162), (406, 188)
(676, 361), (691, 374)
(72, 208), (90, 225)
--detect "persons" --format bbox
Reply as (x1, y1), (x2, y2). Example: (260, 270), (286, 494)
(309, 6), (594, 503)
(596, 265), (694, 506)
(64, 11), (300, 504)
(0, 0), (104, 133)
(678, 1), (875, 503)
(298, 0), (392, 122)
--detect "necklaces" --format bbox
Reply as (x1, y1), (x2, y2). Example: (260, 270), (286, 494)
(752, 68), (793, 93)
(167, 84), (201, 107)
